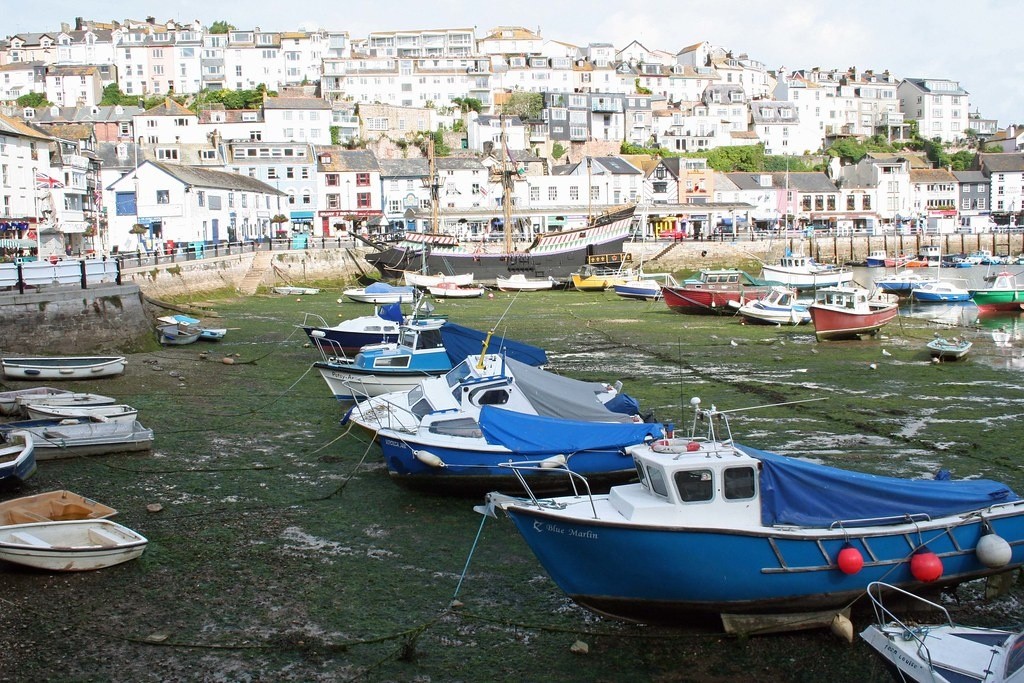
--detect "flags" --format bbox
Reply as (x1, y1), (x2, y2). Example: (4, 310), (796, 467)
(94, 191), (102, 212)
(33, 170), (65, 189)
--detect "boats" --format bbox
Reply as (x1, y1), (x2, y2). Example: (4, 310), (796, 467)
(1, 354), (127, 377)
(302, 297), (446, 353)
(0, 387), (156, 481)
(0, 519), (148, 570)
(0, 490), (121, 525)
(272, 286), (319, 295)
(310, 314), (547, 403)
(857, 580), (1024, 683)
(482, 392), (1024, 627)
(341, 324), (670, 482)
(427, 284), (484, 297)
(499, 247), (1024, 361)
(157, 313), (226, 346)
(343, 282), (422, 304)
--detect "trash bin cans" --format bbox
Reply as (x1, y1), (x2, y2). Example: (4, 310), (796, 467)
(163, 240), (205, 260)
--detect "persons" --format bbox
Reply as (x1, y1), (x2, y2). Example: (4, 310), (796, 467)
(65, 245), (73, 260)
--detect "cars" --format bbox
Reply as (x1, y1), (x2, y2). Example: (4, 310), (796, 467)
(460, 233), (525, 242)
(628, 224), (831, 240)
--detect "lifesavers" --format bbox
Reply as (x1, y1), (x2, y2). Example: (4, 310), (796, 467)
(650, 437), (700, 454)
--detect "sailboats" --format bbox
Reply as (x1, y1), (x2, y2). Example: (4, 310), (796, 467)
(874, 167), (934, 293)
(348, 103), (639, 279)
(762, 154), (854, 285)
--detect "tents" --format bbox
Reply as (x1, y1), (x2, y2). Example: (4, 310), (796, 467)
(0, 238), (38, 263)
